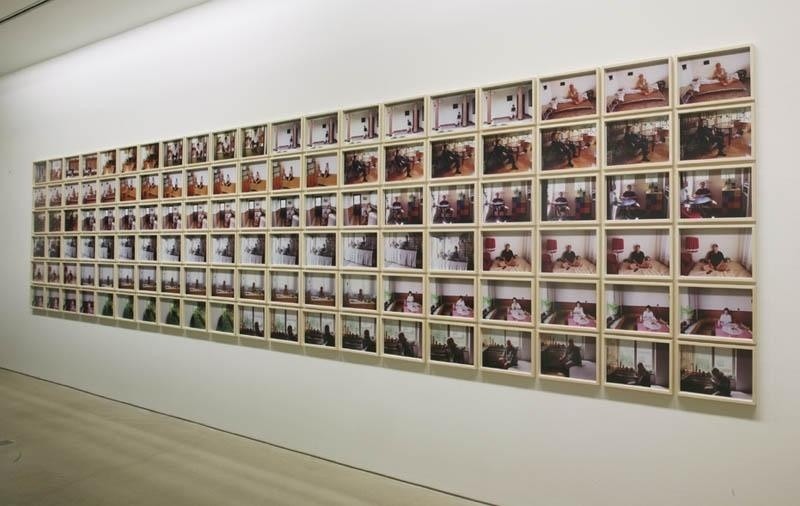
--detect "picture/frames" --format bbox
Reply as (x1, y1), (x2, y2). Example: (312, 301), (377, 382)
(599, 55), (676, 396)
(138, 142), (161, 324)
(340, 101), (382, 357)
(236, 122), (270, 340)
(674, 45), (761, 406)
(93, 147), (117, 321)
(116, 146), (138, 322)
(534, 67), (601, 386)
(208, 129), (239, 334)
(425, 87), (478, 369)
(77, 150), (99, 316)
(62, 155), (83, 314)
(28, 158), (48, 309)
(181, 133), (212, 332)
(160, 136), (187, 328)
(269, 116), (305, 344)
(478, 79), (536, 377)
(379, 95), (428, 363)
(48, 158), (63, 312)
(300, 111), (341, 349)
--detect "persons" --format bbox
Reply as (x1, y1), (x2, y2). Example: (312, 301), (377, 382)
(425, 63), (734, 396)
(30, 153), (138, 319)
(138, 135), (237, 332)
(239, 119), (425, 355)
